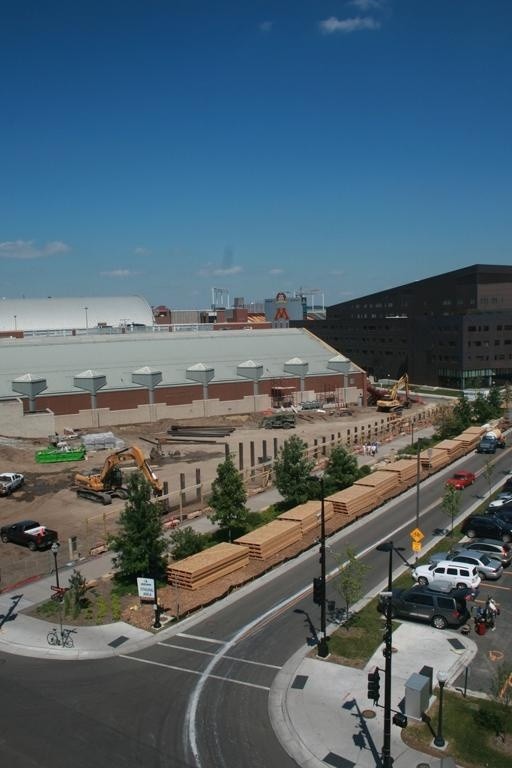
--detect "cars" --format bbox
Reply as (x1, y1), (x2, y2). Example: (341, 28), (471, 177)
(0, 471), (24, 496)
(485, 476), (512, 522)
(446, 469), (476, 490)
(428, 547), (504, 582)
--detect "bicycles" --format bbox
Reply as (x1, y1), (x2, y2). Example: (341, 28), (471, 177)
(46, 626), (76, 647)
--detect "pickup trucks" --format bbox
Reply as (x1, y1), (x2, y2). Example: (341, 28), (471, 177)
(0, 518), (58, 549)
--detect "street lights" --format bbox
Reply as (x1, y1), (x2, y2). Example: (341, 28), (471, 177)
(434, 668), (447, 748)
(411, 421), (416, 446)
(50, 539), (59, 593)
(13, 313), (17, 332)
(304, 477), (329, 658)
(83, 306), (90, 335)
(373, 540), (398, 768)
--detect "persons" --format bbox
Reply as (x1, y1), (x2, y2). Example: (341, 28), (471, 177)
(486, 601), (497, 631)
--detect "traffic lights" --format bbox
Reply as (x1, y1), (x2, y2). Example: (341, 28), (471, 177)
(391, 711), (406, 728)
(309, 575), (322, 605)
(367, 669), (380, 702)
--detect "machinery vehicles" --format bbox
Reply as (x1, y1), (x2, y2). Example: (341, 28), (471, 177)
(149, 436), (183, 465)
(376, 372), (412, 412)
(476, 428), (507, 455)
(34, 438), (87, 464)
(256, 411), (297, 429)
(328, 400), (353, 416)
(72, 445), (169, 515)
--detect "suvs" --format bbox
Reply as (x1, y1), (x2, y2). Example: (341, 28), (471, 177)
(412, 560), (484, 593)
(460, 510), (512, 542)
(378, 585), (469, 631)
(449, 536), (512, 565)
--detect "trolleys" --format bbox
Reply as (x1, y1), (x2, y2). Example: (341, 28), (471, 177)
(471, 604), (495, 631)
